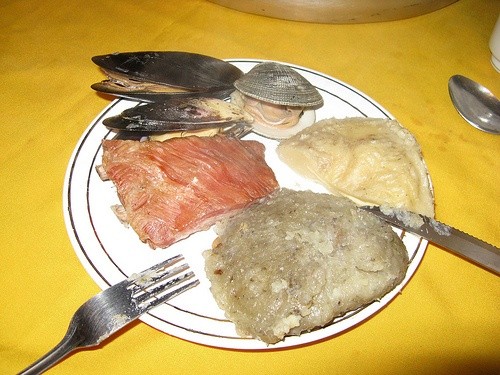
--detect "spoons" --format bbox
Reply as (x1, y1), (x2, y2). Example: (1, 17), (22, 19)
(447, 74), (500, 135)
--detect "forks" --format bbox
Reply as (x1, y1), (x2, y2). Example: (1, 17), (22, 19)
(16, 254), (200, 375)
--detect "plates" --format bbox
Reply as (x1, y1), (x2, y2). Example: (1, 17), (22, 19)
(61, 59), (434, 351)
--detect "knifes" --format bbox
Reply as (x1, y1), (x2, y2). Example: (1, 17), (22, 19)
(360, 204), (500, 274)
